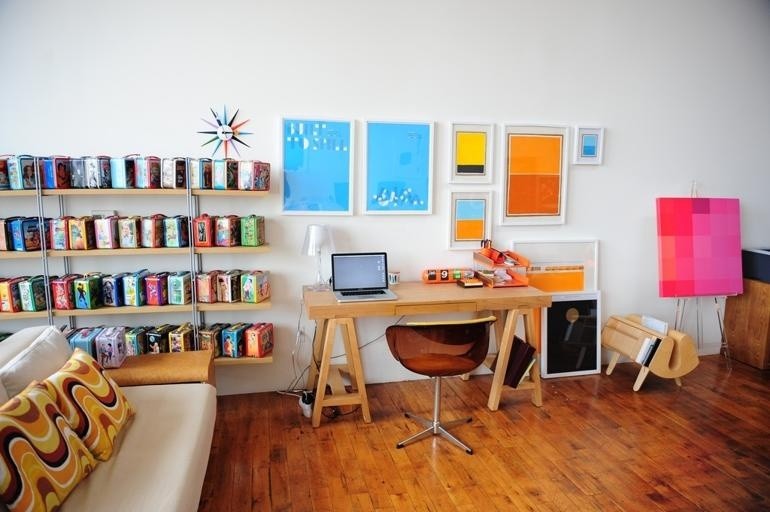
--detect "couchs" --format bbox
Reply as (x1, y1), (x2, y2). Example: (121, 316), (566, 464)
(0, 325), (217, 511)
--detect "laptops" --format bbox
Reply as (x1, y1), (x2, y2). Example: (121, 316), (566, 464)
(331, 252), (401, 302)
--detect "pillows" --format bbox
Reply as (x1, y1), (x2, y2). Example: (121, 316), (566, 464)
(42, 347), (137, 461)
(0, 325), (73, 399)
(0, 380), (102, 512)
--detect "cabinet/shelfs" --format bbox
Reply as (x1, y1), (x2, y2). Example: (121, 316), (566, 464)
(0, 188), (274, 390)
(720, 248), (770, 370)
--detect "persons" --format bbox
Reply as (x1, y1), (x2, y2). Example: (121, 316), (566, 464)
(22, 166), (34, 188)
(172, 338), (180, 352)
(121, 223), (134, 246)
(100, 343), (106, 352)
(78, 283), (88, 308)
(244, 279), (252, 300)
(57, 162), (69, 187)
(150, 342), (160, 355)
(224, 336), (233, 357)
(108, 344), (112, 360)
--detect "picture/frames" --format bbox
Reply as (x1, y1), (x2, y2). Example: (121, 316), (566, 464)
(572, 124), (604, 167)
(279, 114), (355, 216)
(540, 291), (601, 378)
(448, 189), (492, 251)
(364, 121), (434, 216)
(502, 124), (567, 227)
(447, 123), (493, 185)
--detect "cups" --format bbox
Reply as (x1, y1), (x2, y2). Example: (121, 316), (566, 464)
(388, 271), (401, 285)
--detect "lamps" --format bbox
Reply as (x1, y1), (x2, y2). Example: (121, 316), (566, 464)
(303, 224), (337, 292)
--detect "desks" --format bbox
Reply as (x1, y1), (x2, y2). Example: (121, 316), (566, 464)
(101, 348), (215, 386)
(303, 279), (551, 429)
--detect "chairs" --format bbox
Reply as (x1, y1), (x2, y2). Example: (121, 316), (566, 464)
(387, 316), (498, 455)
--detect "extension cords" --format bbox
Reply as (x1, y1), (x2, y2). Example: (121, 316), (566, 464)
(299, 395), (313, 419)
